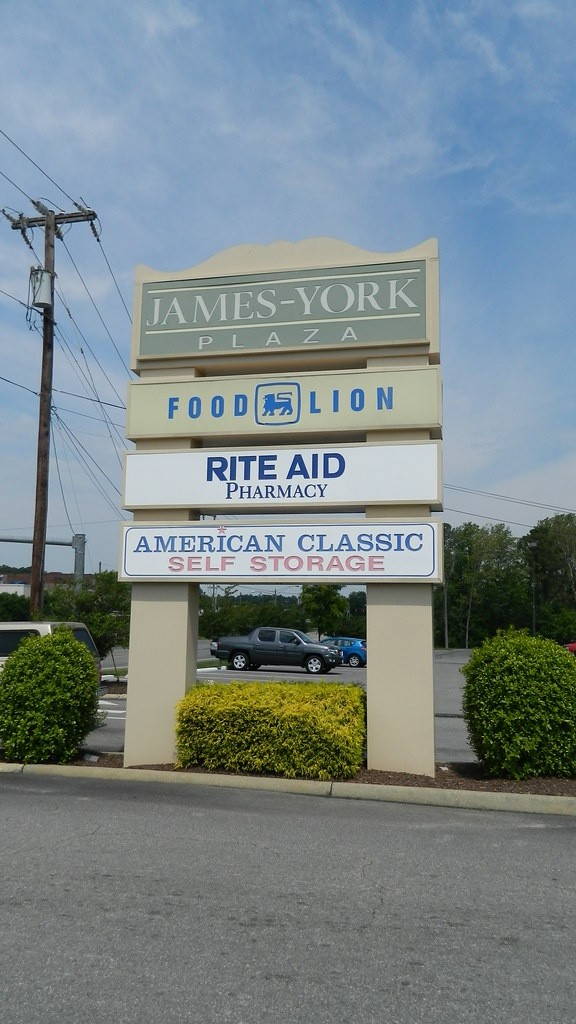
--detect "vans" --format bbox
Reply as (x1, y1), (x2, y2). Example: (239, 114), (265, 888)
(0, 622), (103, 713)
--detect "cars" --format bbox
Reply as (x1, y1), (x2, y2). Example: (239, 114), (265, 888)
(317, 637), (367, 667)
(563, 642), (576, 658)
(209, 625), (344, 673)
